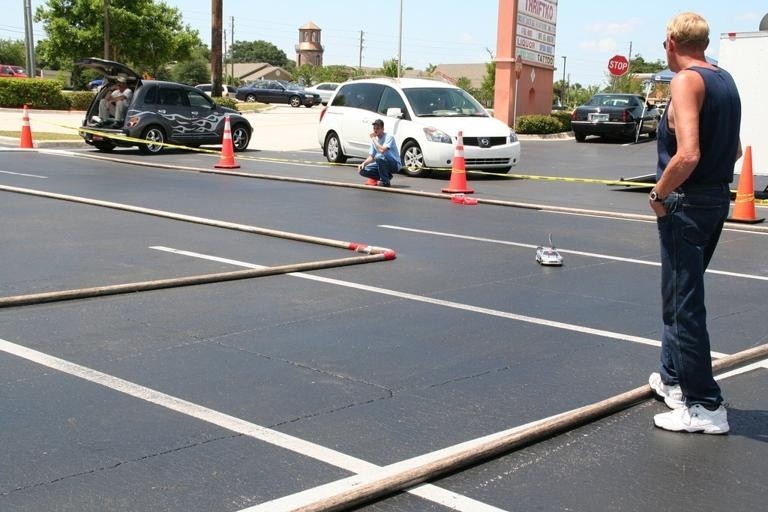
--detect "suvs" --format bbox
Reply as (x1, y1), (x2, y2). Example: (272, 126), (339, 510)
(78, 56), (254, 155)
(0, 64), (38, 80)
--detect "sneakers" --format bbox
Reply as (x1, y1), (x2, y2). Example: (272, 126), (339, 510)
(376, 178), (391, 187)
(110, 120), (125, 129)
(388, 173), (393, 180)
(652, 402), (731, 435)
(648, 371), (688, 410)
(94, 118), (109, 128)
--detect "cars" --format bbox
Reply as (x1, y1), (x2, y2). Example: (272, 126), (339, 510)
(321, 76), (521, 176)
(657, 102), (667, 112)
(571, 94), (661, 143)
(553, 103), (566, 108)
(189, 81), (342, 107)
(88, 78), (104, 92)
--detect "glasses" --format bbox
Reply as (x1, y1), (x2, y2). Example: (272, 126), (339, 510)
(663, 40), (669, 49)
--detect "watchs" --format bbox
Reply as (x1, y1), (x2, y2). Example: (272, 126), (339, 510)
(648, 191), (666, 202)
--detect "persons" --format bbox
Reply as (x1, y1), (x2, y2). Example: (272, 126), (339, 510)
(90, 75), (133, 130)
(648, 12), (743, 434)
(358, 119), (402, 187)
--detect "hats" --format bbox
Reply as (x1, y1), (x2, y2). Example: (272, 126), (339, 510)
(372, 118), (385, 128)
(117, 76), (126, 83)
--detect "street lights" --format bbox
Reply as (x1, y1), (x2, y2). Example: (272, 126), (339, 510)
(346, 30), (363, 71)
(560, 55), (568, 109)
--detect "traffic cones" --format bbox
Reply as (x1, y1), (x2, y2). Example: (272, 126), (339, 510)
(16, 104), (34, 151)
(726, 142), (767, 226)
(441, 130), (475, 197)
(213, 114), (242, 171)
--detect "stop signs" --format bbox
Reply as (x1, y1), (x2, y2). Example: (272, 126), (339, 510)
(607, 55), (629, 76)
